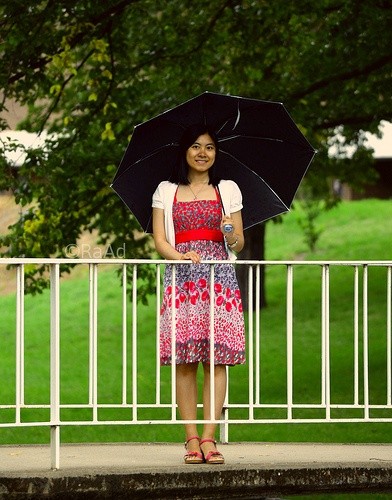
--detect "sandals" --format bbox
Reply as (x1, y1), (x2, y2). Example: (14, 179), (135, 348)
(199, 437), (224, 464)
(183, 436), (203, 464)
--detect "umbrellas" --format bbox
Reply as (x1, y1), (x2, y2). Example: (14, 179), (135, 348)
(110, 90), (318, 235)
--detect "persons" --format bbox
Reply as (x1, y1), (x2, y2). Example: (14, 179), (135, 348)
(152, 126), (245, 466)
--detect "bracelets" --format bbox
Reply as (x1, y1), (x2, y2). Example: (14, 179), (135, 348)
(225, 234), (238, 249)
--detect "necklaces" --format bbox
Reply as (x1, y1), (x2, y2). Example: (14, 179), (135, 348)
(187, 182), (207, 200)
